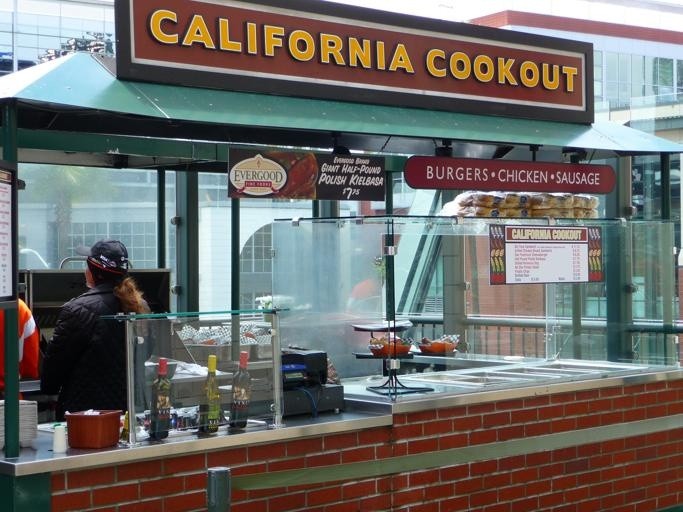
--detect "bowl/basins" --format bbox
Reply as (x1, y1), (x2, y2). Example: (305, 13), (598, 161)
(418, 343), (457, 353)
(368, 343), (411, 356)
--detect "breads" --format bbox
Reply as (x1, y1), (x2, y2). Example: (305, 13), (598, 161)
(457, 194), (597, 219)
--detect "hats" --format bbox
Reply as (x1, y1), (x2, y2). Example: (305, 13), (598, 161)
(75, 240), (129, 274)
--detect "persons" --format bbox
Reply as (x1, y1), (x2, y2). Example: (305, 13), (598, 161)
(41, 239), (156, 422)
(0, 299), (41, 401)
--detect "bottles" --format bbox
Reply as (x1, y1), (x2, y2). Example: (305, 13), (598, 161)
(51, 425), (68, 454)
(229, 351), (251, 429)
(149, 357), (172, 440)
(199, 354), (221, 433)
(119, 412), (130, 442)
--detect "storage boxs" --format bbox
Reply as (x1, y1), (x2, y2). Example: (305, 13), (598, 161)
(64, 410), (122, 448)
